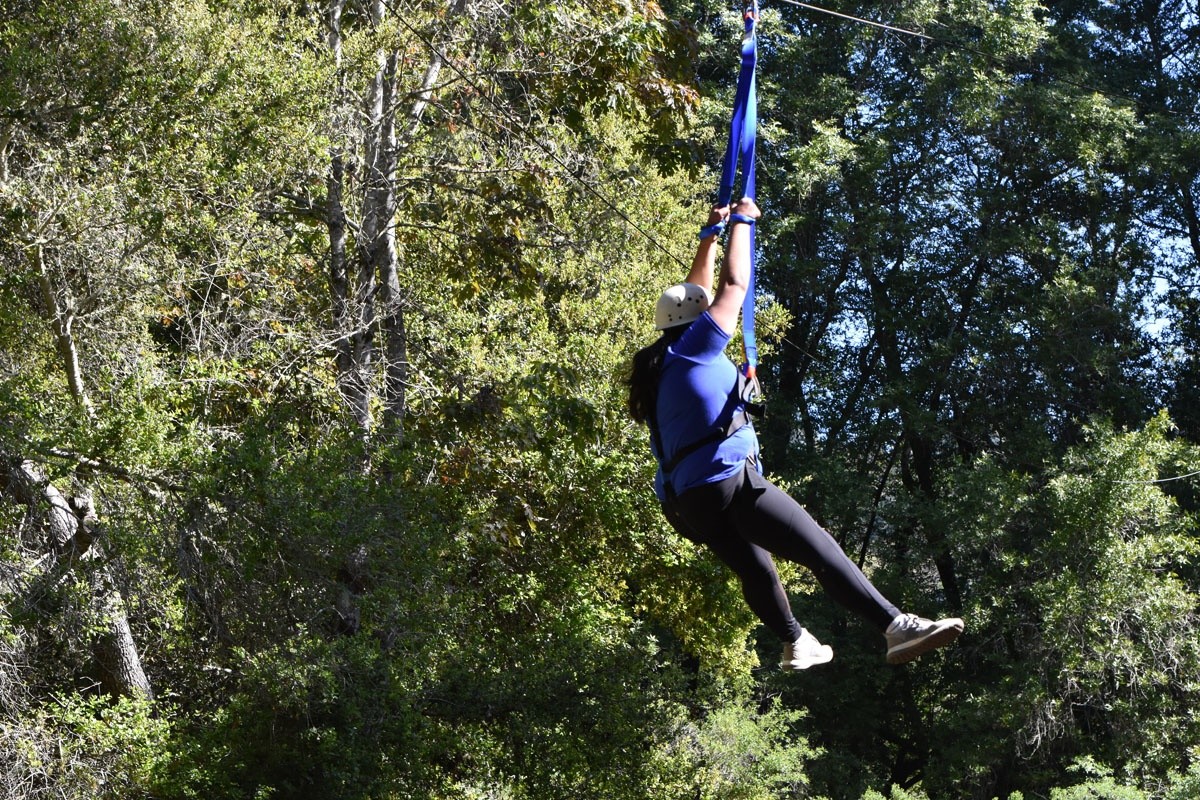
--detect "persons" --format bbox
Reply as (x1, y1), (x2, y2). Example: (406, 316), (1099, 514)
(628, 198), (963, 670)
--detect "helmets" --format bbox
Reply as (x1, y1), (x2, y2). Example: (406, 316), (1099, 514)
(656, 282), (711, 331)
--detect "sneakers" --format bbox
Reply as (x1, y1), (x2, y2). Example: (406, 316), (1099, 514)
(781, 628), (833, 672)
(885, 613), (965, 665)
